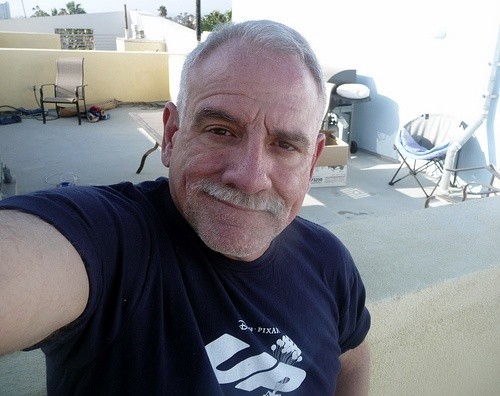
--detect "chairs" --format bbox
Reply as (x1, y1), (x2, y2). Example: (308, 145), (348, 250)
(388, 113), (467, 198)
(39, 57), (88, 126)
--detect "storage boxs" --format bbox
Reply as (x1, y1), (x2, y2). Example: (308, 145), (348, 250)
(310, 130), (351, 187)
